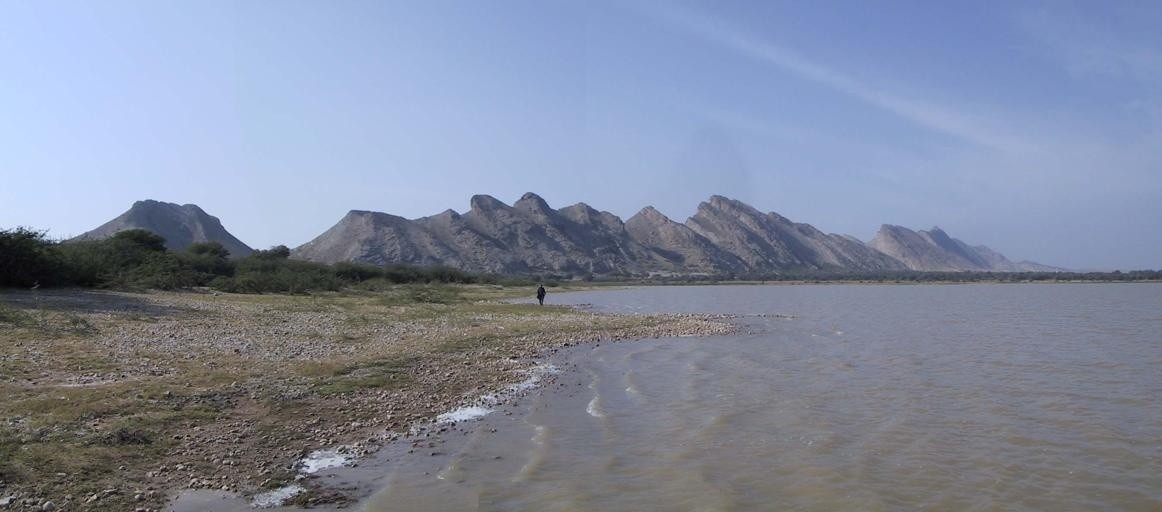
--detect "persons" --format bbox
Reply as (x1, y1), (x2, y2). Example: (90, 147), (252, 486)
(537, 283), (547, 306)
